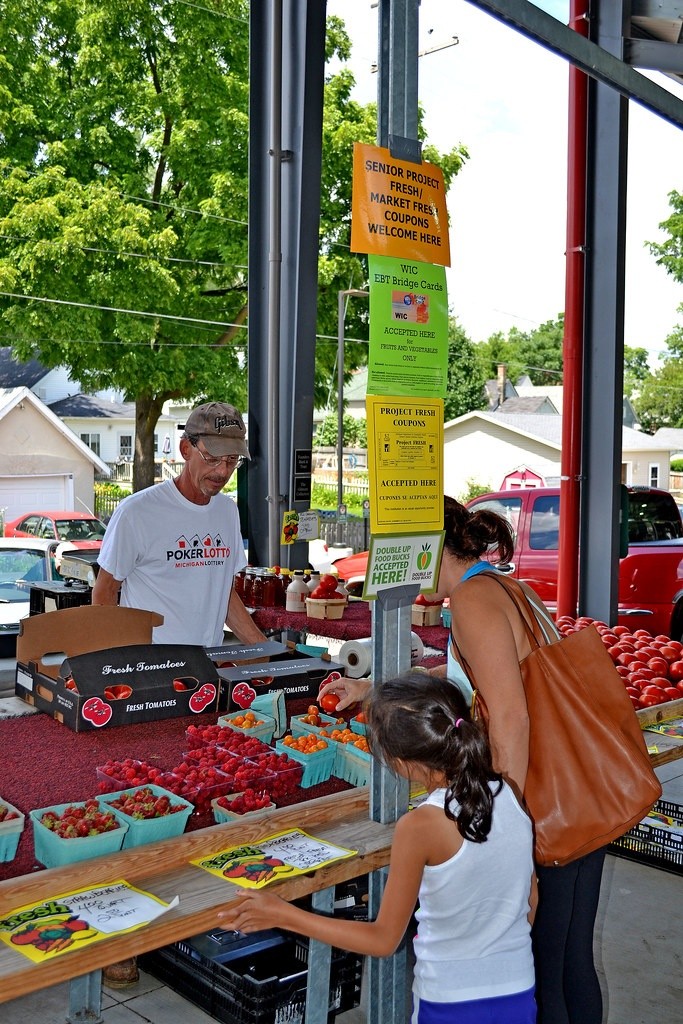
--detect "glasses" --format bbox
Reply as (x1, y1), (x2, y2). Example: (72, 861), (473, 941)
(195, 444), (243, 469)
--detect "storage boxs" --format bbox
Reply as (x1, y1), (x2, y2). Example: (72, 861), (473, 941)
(0, 597), (371, 867)
(281, 872), (418, 1011)
(410, 600), (441, 625)
(135, 926), (343, 1024)
(607, 798), (683, 875)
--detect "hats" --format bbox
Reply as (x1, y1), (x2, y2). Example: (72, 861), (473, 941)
(185, 402), (251, 461)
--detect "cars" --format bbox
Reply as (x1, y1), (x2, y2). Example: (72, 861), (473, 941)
(0, 535), (62, 640)
(2, 508), (107, 550)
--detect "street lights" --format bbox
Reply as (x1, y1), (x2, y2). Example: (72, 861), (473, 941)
(336, 287), (371, 508)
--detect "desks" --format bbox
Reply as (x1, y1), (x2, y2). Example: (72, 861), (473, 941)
(0, 790), (431, 1024)
(244, 603), (683, 770)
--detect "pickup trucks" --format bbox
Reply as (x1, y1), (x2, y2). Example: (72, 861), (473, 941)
(333, 482), (683, 646)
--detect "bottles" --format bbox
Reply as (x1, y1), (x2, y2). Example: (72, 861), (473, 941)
(232, 565), (324, 612)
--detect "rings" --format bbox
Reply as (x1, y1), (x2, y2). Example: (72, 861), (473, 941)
(333, 692), (335, 695)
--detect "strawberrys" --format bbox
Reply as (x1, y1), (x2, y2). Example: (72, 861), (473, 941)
(40, 787), (187, 840)
(0, 802), (20, 823)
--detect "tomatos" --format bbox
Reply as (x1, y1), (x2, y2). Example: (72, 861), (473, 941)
(550, 615), (683, 711)
(65, 678), (186, 700)
(224, 693), (370, 755)
(218, 661), (274, 686)
(414, 594), (444, 607)
(310, 573), (343, 599)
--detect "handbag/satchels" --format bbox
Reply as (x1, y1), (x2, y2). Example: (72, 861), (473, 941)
(450, 572), (662, 867)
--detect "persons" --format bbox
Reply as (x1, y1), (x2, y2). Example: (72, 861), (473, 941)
(16, 545), (66, 589)
(91, 402), (269, 647)
(216, 668), (539, 1024)
(316, 496), (608, 1024)
